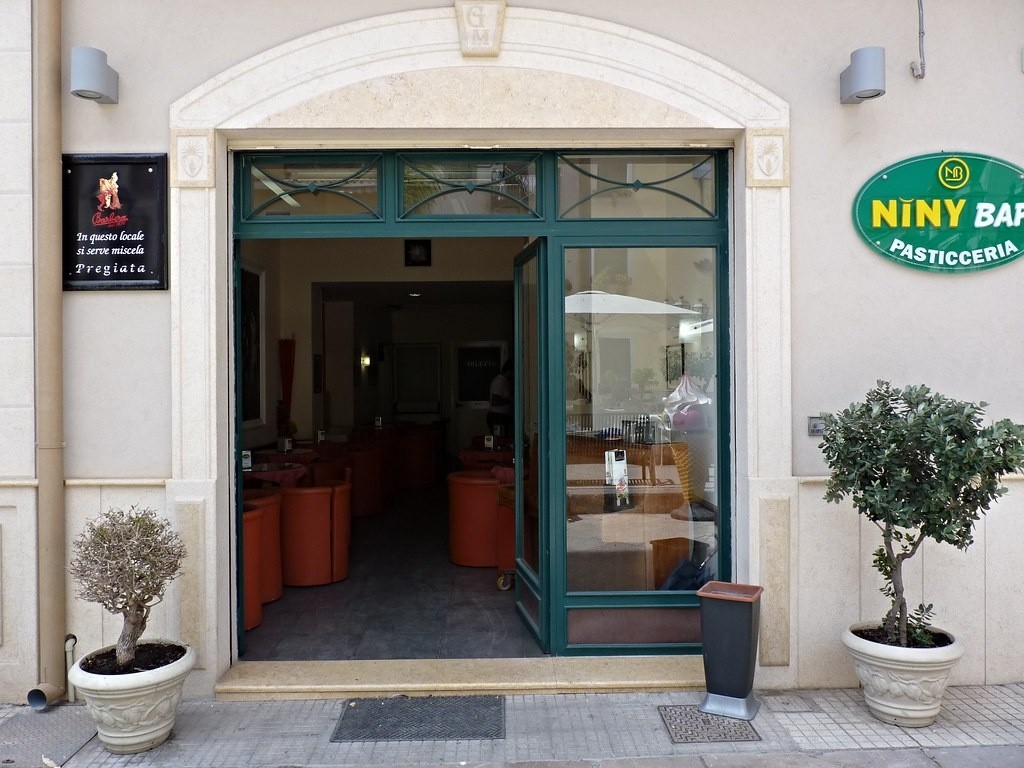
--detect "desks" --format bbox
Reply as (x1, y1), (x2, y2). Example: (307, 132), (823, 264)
(350, 428), (397, 440)
(244, 461), (309, 487)
(254, 447), (316, 463)
(299, 442), (347, 453)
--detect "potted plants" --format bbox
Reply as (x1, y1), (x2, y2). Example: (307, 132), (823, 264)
(67, 502), (200, 755)
(818, 379), (1024, 729)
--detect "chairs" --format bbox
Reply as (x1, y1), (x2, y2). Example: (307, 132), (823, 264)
(243, 420), (514, 633)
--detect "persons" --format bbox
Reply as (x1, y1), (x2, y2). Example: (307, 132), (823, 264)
(487, 359), (515, 438)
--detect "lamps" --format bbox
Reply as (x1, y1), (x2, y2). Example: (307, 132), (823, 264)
(70, 46), (119, 104)
(673, 295), (691, 310)
(840, 45), (886, 105)
(692, 298), (710, 321)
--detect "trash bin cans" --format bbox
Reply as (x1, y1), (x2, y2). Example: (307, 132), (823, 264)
(696, 580), (765, 723)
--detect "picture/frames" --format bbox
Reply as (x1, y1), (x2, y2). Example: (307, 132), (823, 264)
(240, 258), (267, 429)
(665, 342), (684, 391)
